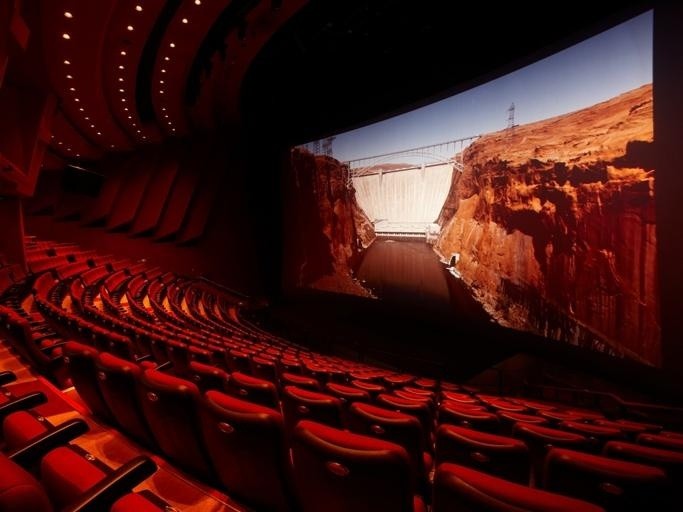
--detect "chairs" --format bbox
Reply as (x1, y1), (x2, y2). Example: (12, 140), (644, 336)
(0, 237), (683, 511)
